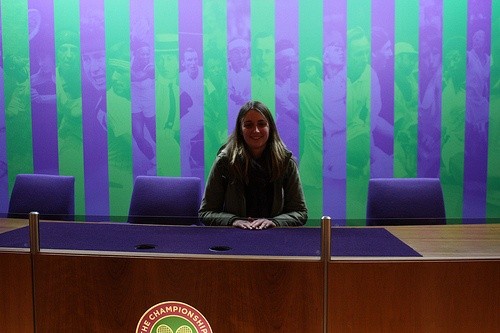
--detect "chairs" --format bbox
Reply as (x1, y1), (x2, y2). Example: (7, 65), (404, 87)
(127, 175), (202, 225)
(366, 178), (447, 225)
(7, 174), (75, 222)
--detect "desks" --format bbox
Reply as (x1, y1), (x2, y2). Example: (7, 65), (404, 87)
(0, 217), (500, 333)
